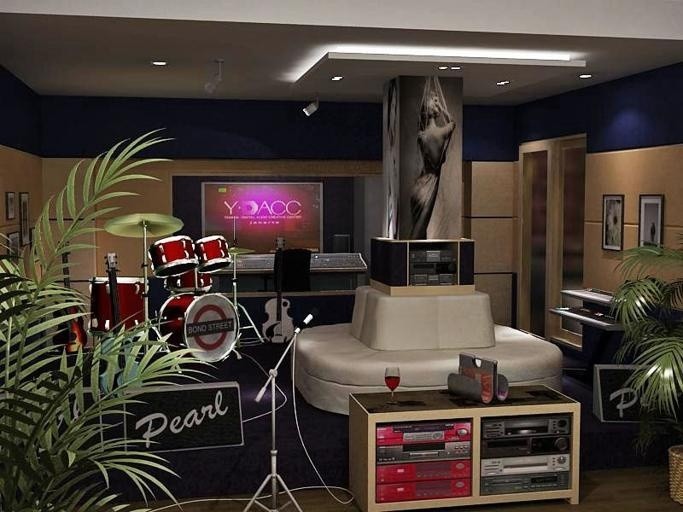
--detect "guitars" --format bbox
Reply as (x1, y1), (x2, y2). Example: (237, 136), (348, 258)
(54, 240), (87, 352)
(262, 236), (295, 343)
(100, 252), (141, 396)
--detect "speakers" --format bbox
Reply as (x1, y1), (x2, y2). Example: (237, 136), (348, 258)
(35, 387), (104, 460)
(592, 364), (683, 424)
(123, 381), (245, 454)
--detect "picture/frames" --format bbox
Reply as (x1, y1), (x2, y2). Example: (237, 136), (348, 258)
(7, 231), (19, 261)
(638, 194), (664, 257)
(5, 191), (15, 221)
(18, 192), (30, 247)
(602, 194), (624, 251)
(30, 227), (37, 255)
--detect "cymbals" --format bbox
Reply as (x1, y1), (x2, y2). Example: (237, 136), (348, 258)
(104, 213), (185, 238)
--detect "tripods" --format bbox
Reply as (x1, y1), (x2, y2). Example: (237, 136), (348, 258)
(243, 332), (304, 512)
(232, 255), (265, 343)
(141, 225), (183, 374)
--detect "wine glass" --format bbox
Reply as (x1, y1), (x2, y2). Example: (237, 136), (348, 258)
(383, 366), (401, 405)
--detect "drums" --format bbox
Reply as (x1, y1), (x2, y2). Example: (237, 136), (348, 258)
(163, 269), (213, 292)
(90, 275), (149, 333)
(150, 236), (199, 279)
(197, 235), (232, 275)
(158, 292), (240, 363)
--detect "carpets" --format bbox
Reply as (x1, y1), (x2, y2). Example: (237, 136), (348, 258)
(55, 350), (683, 504)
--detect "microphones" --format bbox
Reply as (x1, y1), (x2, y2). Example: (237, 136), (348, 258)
(294, 314), (314, 335)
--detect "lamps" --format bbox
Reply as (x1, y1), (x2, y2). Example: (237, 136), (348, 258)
(202, 58), (227, 96)
(303, 92), (319, 116)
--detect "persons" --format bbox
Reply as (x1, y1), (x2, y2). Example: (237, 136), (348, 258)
(409, 91), (456, 239)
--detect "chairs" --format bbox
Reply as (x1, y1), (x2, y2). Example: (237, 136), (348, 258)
(290, 286), (563, 416)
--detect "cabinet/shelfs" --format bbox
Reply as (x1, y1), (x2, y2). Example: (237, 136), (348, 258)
(547, 290), (627, 332)
(348, 384), (581, 512)
